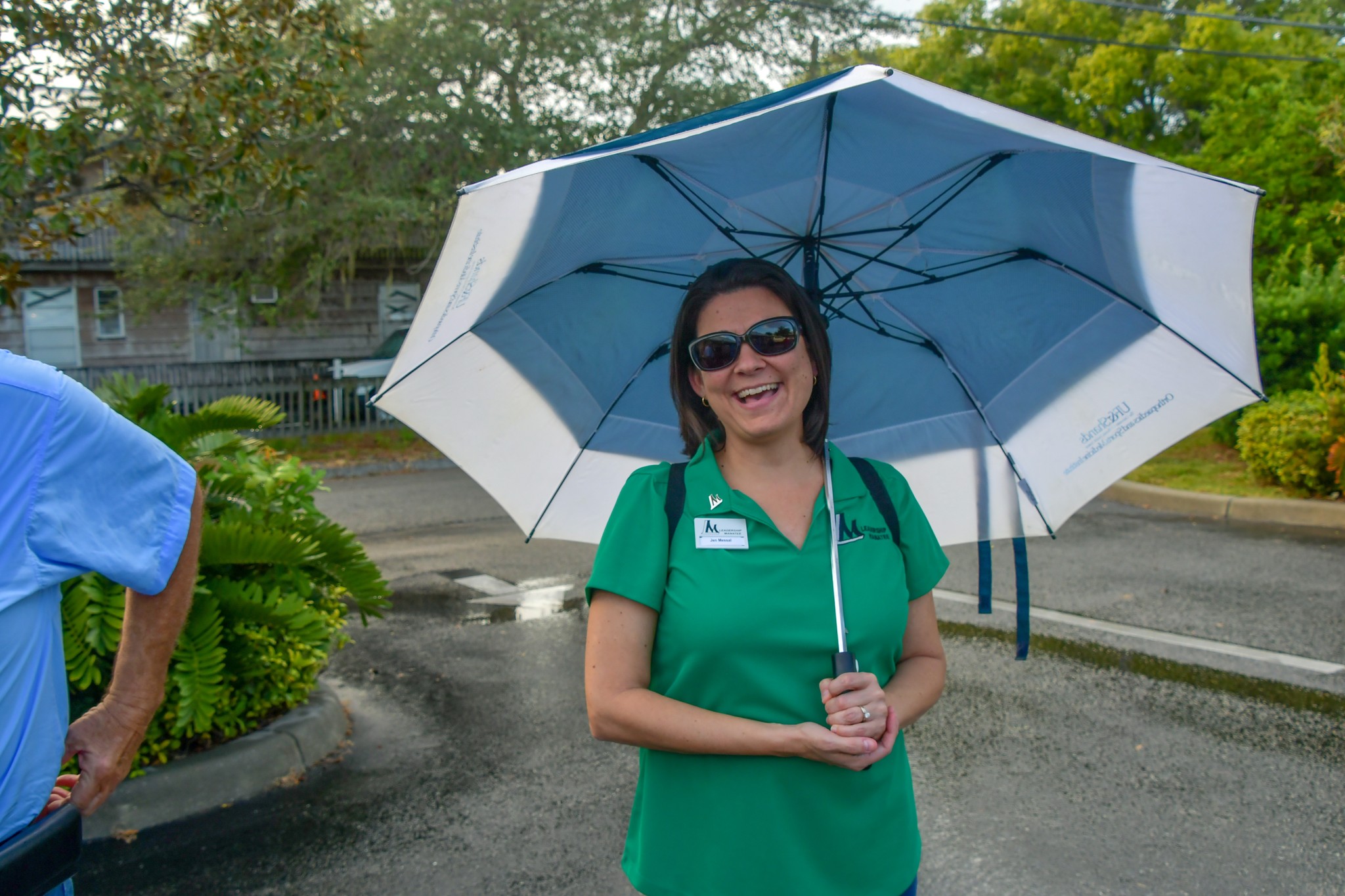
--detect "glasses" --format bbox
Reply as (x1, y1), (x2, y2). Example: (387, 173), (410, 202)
(687, 316), (805, 372)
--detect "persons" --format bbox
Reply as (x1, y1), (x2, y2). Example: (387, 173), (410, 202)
(0, 343), (206, 896)
(580, 257), (952, 893)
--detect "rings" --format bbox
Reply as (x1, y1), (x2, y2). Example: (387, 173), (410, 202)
(858, 705), (871, 723)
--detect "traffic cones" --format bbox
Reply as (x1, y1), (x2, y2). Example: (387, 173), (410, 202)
(312, 374), (326, 402)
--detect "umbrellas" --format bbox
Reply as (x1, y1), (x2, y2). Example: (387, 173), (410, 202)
(361, 62), (1269, 701)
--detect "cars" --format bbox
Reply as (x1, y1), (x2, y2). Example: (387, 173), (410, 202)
(326, 326), (408, 426)
